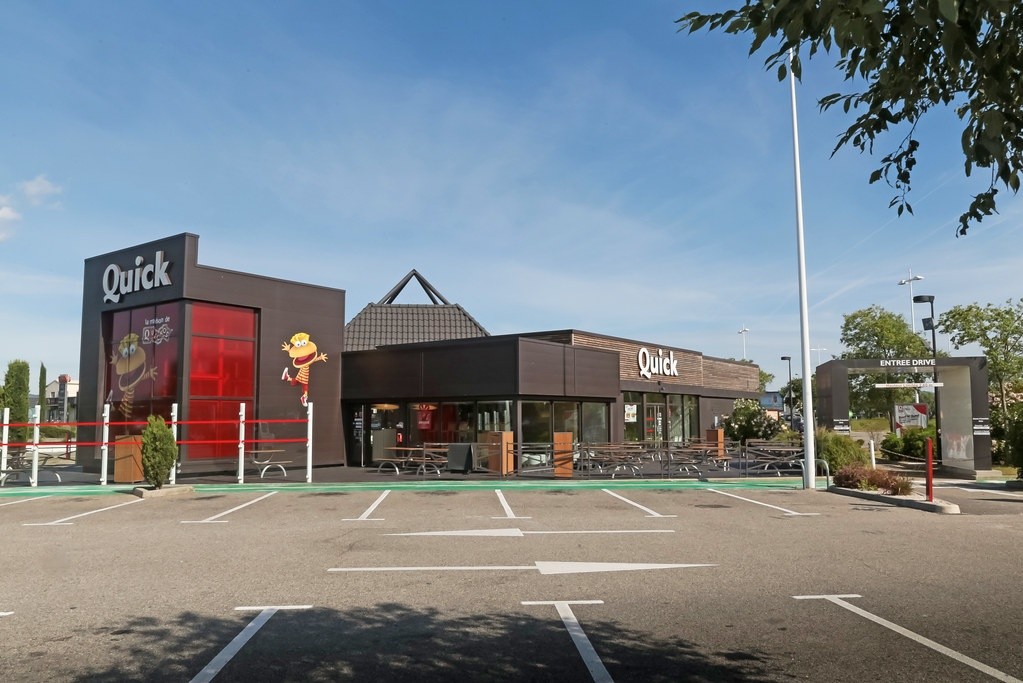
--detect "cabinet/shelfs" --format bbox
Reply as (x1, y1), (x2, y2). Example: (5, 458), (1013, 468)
(554, 432), (573, 478)
(114, 435), (145, 484)
(707, 429), (724, 456)
(489, 431), (513, 475)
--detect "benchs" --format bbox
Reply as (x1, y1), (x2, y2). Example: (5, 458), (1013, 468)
(376, 458), (449, 476)
(1, 469), (64, 486)
(254, 460), (293, 480)
(577, 457), (733, 479)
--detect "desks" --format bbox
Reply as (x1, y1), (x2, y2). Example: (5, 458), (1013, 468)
(384, 447), (449, 474)
(246, 450), (285, 473)
(583, 442), (730, 479)
